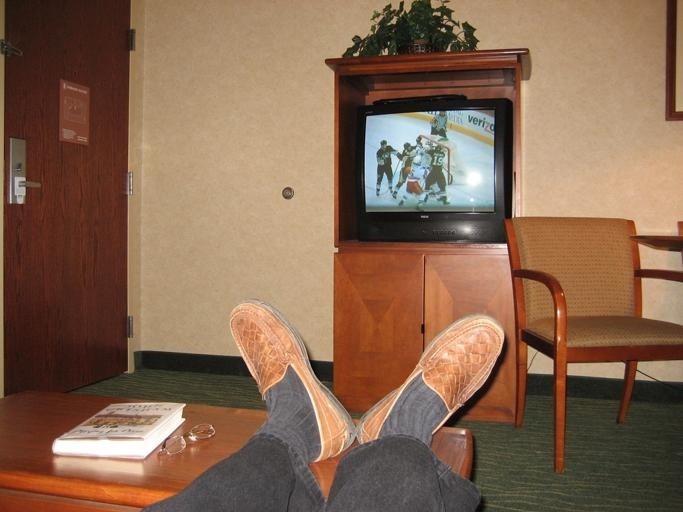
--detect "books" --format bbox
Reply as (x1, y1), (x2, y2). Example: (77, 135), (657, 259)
(52, 401), (188, 461)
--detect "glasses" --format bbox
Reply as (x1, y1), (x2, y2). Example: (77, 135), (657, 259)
(156, 422), (216, 457)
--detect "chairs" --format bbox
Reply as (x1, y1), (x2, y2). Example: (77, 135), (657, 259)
(504, 217), (683, 475)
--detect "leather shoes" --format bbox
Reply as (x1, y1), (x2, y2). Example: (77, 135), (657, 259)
(228, 298), (356, 462)
(357, 313), (505, 448)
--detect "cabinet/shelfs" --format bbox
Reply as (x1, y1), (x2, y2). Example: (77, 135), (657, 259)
(323, 47), (532, 426)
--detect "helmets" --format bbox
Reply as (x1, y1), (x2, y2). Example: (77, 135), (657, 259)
(380, 140), (388, 146)
(403, 136), (444, 156)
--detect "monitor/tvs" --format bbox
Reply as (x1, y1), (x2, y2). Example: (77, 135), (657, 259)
(356, 95), (512, 243)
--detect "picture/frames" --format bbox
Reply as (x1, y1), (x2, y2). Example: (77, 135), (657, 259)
(665, 0), (683, 121)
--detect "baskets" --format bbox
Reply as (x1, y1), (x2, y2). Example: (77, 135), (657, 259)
(396, 39), (445, 55)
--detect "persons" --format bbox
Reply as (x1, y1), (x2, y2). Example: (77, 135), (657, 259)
(137, 295), (509, 512)
(374, 112), (450, 208)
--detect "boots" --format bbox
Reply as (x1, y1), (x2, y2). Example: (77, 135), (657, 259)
(392, 191), (397, 199)
(376, 189), (380, 197)
(417, 192), (449, 208)
(399, 200), (404, 207)
(389, 186), (392, 193)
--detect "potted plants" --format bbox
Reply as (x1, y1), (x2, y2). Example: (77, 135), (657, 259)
(341, 0), (480, 58)
